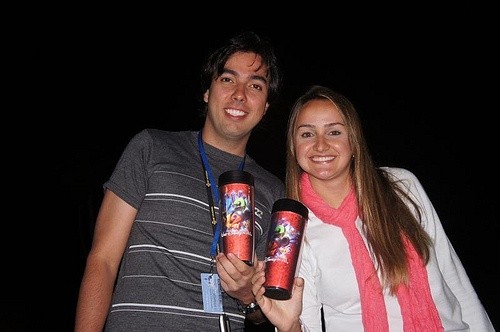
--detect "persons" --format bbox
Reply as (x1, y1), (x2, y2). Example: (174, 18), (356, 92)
(249, 83), (499, 331)
(74, 26), (287, 332)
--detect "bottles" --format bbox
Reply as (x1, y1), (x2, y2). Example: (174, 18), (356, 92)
(218, 169), (259, 268)
(263, 198), (311, 302)
